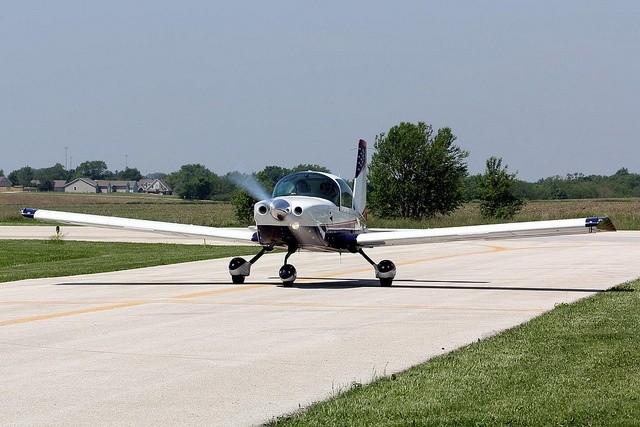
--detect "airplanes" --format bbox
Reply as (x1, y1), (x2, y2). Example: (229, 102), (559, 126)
(19, 139), (617, 287)
